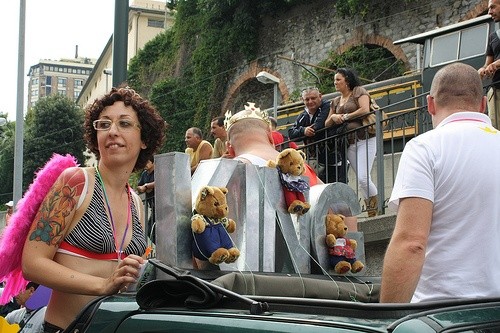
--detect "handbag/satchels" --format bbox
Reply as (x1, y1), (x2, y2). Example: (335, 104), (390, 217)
(362, 98), (390, 133)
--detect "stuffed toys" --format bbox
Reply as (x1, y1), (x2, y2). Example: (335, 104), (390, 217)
(191, 186), (241, 264)
(326, 214), (364, 274)
(269, 148), (310, 216)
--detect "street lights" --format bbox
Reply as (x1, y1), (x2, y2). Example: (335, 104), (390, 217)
(256, 70), (280, 121)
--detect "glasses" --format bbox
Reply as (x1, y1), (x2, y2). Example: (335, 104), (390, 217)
(302, 86), (316, 91)
(93, 118), (138, 131)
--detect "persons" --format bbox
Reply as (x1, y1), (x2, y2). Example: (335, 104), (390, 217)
(288, 86), (349, 187)
(378, 63), (500, 303)
(21, 87), (169, 333)
(0, 102), (357, 333)
(325, 68), (383, 217)
(477, 0), (500, 130)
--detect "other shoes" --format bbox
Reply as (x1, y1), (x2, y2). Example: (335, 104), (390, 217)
(367, 204), (380, 217)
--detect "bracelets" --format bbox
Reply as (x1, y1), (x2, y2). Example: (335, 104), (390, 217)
(343, 114), (348, 120)
(145, 183), (147, 188)
